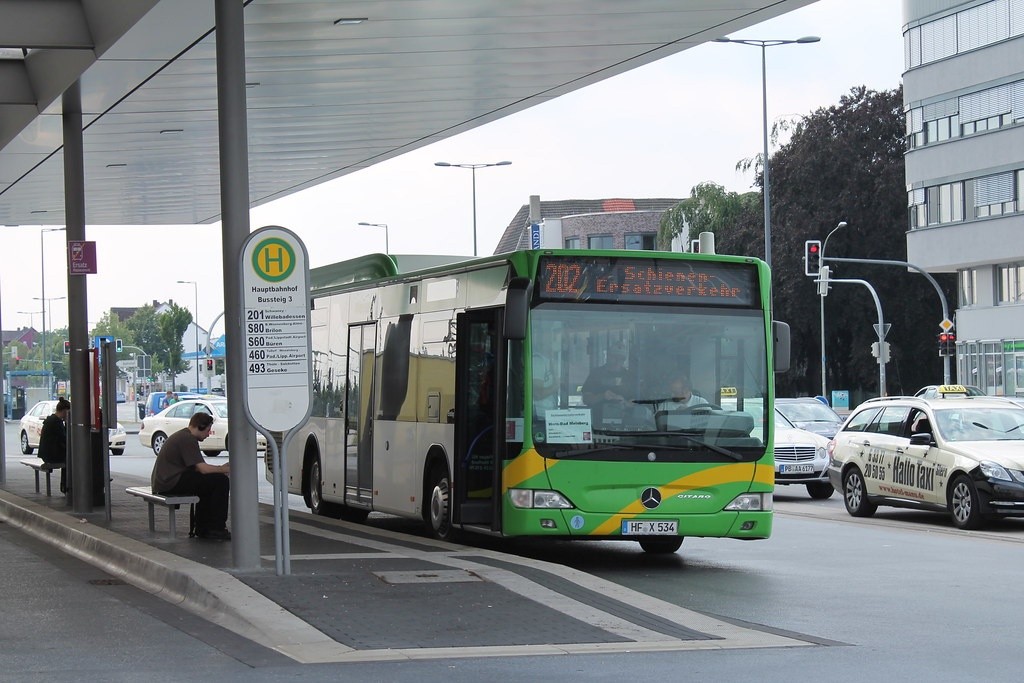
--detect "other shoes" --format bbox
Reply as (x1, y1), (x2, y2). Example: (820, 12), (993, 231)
(194, 527), (232, 540)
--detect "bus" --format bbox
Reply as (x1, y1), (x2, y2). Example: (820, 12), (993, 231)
(264, 247), (790, 555)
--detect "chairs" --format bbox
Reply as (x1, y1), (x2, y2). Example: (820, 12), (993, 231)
(174, 409), (183, 416)
(888, 416), (902, 435)
(915, 419), (935, 442)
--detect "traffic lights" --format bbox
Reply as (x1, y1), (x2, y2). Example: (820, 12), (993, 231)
(206, 359), (214, 371)
(804, 239), (822, 276)
(820, 266), (833, 296)
(116, 339), (124, 353)
(947, 333), (956, 356)
(64, 340), (71, 355)
(939, 332), (948, 357)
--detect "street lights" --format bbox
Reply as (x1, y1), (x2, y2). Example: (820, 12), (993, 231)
(17, 311), (49, 358)
(40, 226), (67, 373)
(433, 161), (514, 255)
(176, 280), (200, 394)
(357, 222), (391, 254)
(33, 296), (67, 386)
(817, 220), (850, 400)
(710, 36), (824, 274)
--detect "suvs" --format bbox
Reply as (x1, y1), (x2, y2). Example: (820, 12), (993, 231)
(827, 395), (1024, 531)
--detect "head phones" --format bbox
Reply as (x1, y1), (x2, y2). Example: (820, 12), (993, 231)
(198, 417), (213, 431)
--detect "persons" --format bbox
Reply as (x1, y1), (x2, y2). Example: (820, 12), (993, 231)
(180, 384), (186, 392)
(468, 345), (490, 415)
(163, 391), (184, 410)
(151, 413), (230, 540)
(533, 330), (561, 420)
(654, 374), (712, 416)
(37, 397), (70, 494)
(581, 342), (636, 427)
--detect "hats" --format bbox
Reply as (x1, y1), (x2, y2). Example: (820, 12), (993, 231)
(608, 340), (627, 361)
(173, 393), (178, 396)
(165, 391), (173, 396)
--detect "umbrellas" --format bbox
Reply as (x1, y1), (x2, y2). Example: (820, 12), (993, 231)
(180, 334), (227, 397)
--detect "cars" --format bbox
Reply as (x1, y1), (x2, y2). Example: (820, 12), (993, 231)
(116, 392), (127, 403)
(211, 387), (226, 397)
(19, 400), (126, 457)
(912, 384), (987, 400)
(717, 395), (849, 501)
(138, 399), (268, 459)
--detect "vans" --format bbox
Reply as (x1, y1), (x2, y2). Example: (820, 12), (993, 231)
(189, 388), (208, 395)
(143, 390), (200, 418)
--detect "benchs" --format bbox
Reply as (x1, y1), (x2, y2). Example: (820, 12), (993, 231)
(126, 486), (200, 539)
(19, 458), (66, 496)
